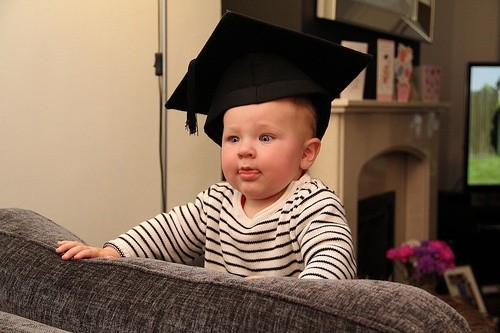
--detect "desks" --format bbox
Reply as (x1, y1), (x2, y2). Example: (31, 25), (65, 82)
(285, 102), (451, 260)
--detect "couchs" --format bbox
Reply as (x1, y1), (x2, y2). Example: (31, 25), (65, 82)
(0, 204), (472, 333)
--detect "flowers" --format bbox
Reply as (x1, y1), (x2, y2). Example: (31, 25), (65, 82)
(387, 240), (455, 288)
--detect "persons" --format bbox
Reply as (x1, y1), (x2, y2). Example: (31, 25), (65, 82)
(56, 10), (373, 281)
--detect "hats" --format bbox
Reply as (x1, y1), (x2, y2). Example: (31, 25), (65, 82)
(164, 10), (375, 147)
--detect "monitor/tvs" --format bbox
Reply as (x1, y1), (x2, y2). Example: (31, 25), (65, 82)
(464, 63), (500, 188)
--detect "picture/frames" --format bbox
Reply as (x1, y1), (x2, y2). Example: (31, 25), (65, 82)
(464, 62), (500, 191)
